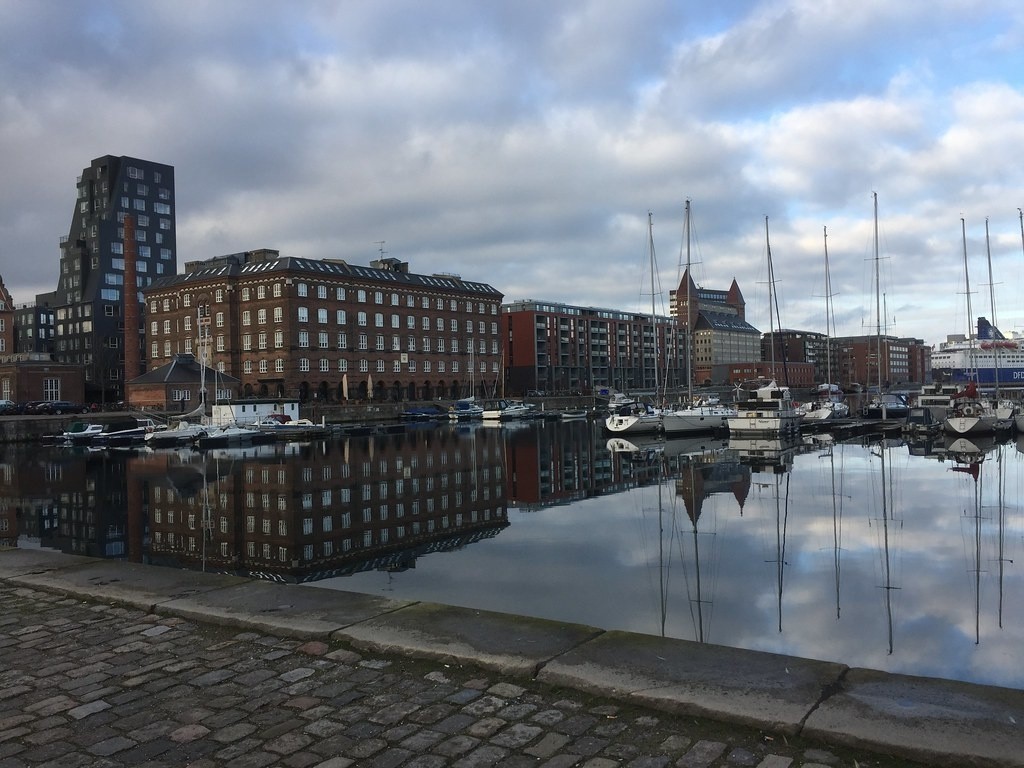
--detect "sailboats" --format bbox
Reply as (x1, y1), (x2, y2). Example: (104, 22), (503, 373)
(663, 197), (740, 432)
(605, 429), (732, 643)
(448, 337), (529, 418)
(945, 434), (996, 645)
(728, 432), (804, 632)
(603, 212), (676, 432)
(725, 215), (806, 431)
(794, 191), (1024, 435)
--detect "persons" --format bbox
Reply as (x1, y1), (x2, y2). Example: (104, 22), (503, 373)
(180, 397), (185, 412)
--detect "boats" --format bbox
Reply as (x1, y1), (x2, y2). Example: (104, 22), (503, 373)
(40, 419), (260, 445)
(397, 407), (448, 421)
(237, 413), (332, 437)
(547, 411), (588, 418)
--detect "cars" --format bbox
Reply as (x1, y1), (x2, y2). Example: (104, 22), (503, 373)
(347, 391), (370, 405)
(0, 400), (89, 415)
(528, 389), (546, 396)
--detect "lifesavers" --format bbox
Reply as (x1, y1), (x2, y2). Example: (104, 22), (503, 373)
(962, 406), (974, 416)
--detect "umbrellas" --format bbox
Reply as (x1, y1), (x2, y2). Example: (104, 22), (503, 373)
(367, 374), (374, 403)
